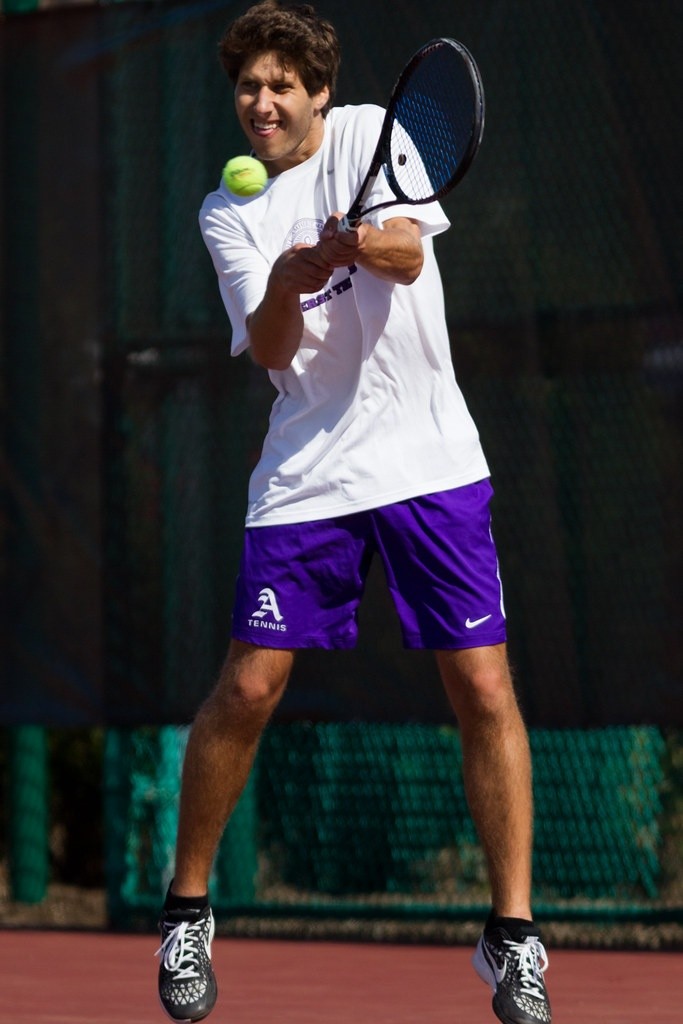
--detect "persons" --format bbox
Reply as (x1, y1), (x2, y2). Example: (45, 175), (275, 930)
(154, 0), (552, 1024)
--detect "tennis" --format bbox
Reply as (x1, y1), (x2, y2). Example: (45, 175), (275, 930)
(223, 155), (268, 197)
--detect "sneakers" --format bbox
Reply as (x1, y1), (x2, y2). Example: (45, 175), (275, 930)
(155, 904), (218, 1024)
(470, 909), (555, 1024)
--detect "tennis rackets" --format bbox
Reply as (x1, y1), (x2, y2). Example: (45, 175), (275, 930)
(334, 35), (487, 235)
